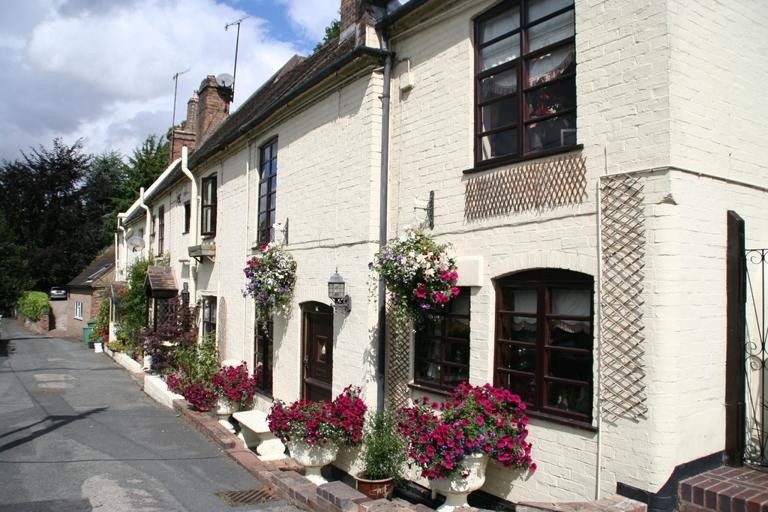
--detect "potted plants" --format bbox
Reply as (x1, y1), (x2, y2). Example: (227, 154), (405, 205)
(350, 406), (413, 502)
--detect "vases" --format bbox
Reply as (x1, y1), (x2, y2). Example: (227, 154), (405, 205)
(209, 389), (240, 421)
(410, 452), (490, 512)
(284, 436), (339, 486)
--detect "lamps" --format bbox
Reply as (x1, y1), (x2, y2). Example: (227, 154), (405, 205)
(328, 266), (351, 314)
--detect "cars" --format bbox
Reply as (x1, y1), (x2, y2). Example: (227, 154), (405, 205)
(50, 287), (67, 302)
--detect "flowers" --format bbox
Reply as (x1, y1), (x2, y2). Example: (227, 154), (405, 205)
(209, 360), (257, 410)
(397, 382), (537, 481)
(240, 241), (298, 343)
(264, 385), (368, 451)
(364, 229), (460, 335)
